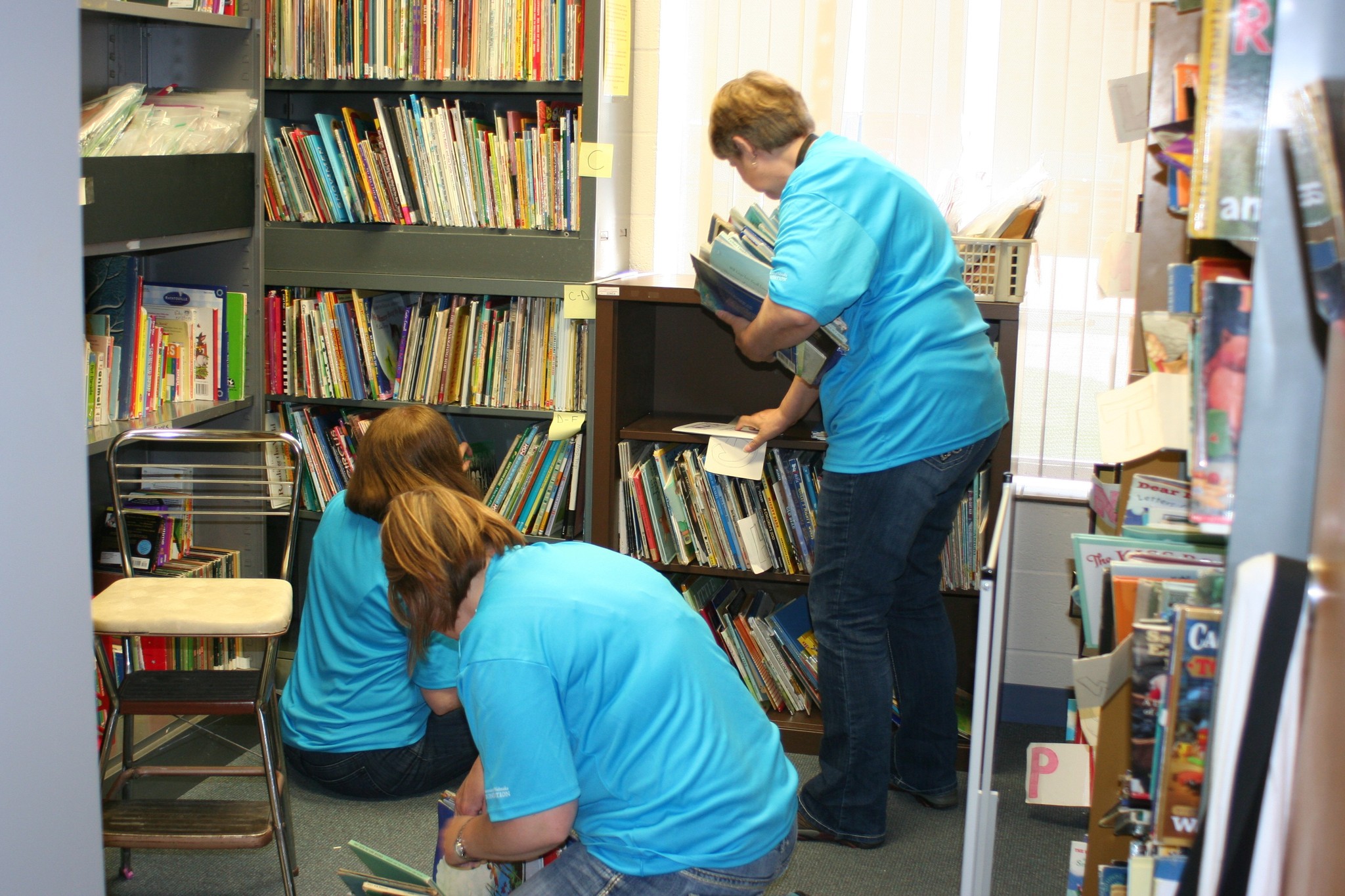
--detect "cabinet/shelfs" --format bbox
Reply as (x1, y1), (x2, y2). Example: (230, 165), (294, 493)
(161, 2), (637, 702)
(79, 0), (261, 780)
(593, 272), (1017, 770)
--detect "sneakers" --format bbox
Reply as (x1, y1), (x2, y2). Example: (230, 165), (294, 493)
(795, 785), (884, 849)
(888, 778), (958, 808)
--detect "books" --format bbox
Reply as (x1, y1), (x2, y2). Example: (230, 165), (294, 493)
(259, 92), (583, 232)
(689, 201), (853, 390)
(1124, 0), (1282, 247)
(662, 566), (976, 746)
(152, 0), (243, 19)
(259, 285), (590, 417)
(94, 451), (250, 758)
(83, 249), (249, 427)
(608, 440), (989, 597)
(1136, 244), (1261, 539)
(260, 398), (587, 541)
(263, 1), (584, 83)
(1054, 459), (1235, 893)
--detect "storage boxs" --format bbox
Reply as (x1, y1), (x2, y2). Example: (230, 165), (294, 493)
(952, 236), (1033, 303)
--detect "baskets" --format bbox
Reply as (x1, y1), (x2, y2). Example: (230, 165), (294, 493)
(950, 233), (1035, 303)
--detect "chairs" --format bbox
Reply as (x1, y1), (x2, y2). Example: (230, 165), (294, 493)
(90, 430), (306, 895)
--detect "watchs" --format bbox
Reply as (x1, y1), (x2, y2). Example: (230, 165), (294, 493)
(454, 815), (485, 863)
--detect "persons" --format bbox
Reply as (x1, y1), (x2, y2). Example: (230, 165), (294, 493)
(707, 69), (1015, 851)
(377, 483), (804, 894)
(272, 401), (479, 804)
